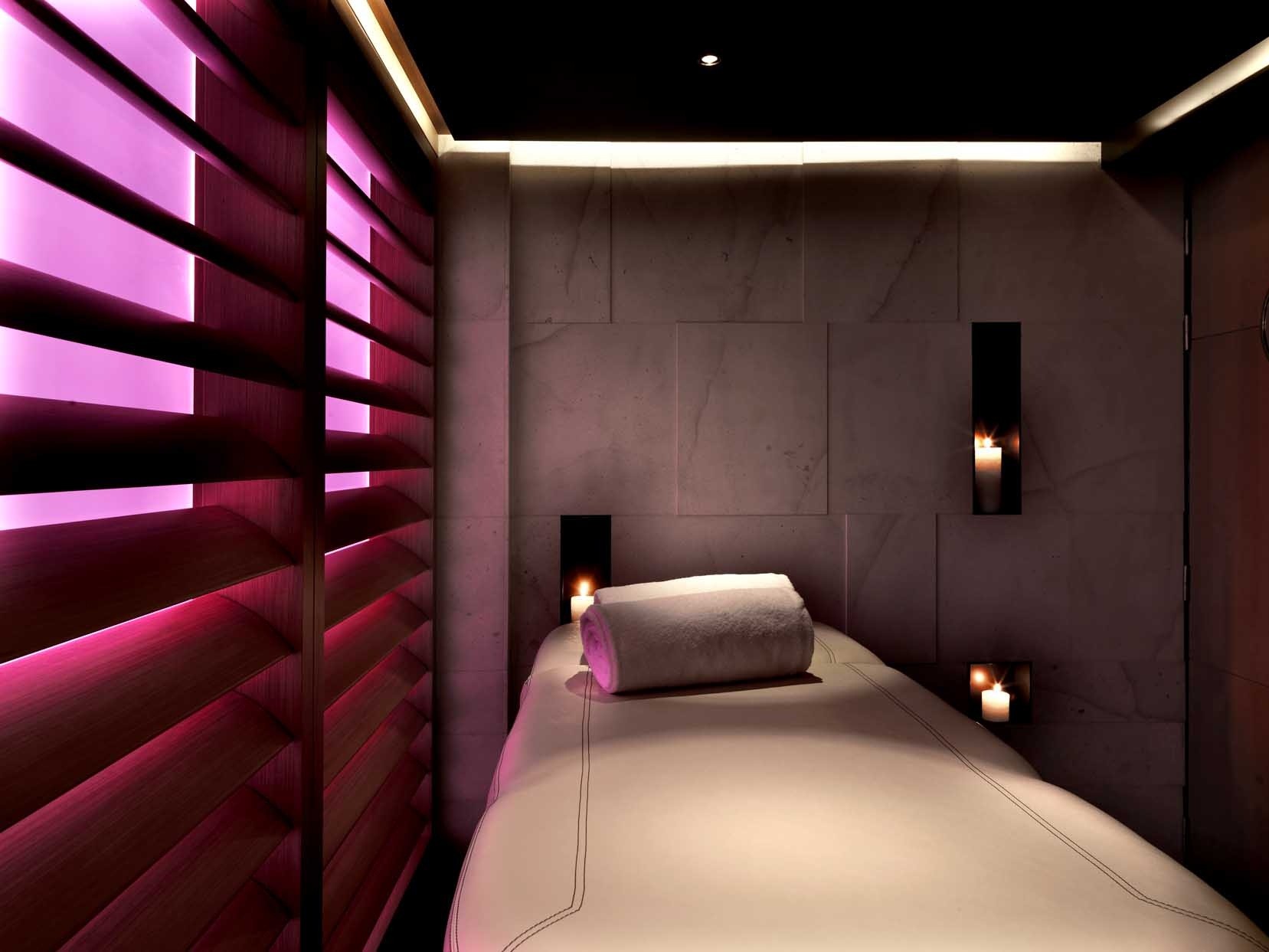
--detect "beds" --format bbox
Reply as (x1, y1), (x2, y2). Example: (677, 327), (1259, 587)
(442, 570), (1269, 952)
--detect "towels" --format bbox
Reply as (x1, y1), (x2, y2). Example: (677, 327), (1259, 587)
(579, 572), (814, 695)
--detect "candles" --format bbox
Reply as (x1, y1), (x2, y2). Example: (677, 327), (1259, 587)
(975, 436), (1002, 515)
(981, 683), (1010, 723)
(571, 576), (595, 622)
